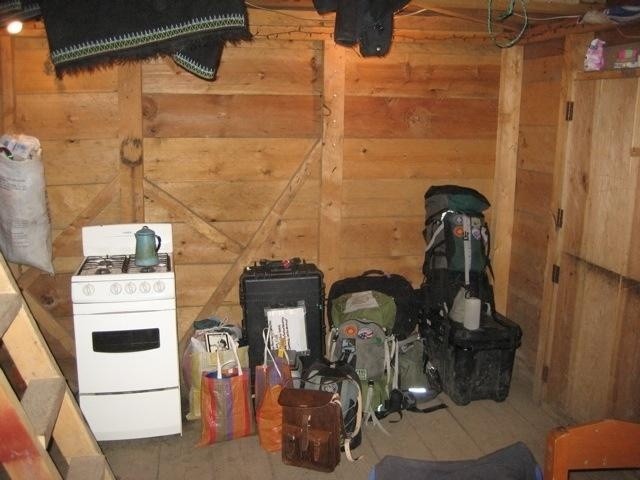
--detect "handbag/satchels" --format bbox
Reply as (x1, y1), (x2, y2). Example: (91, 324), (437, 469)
(182, 318), (304, 453)
(323, 267), (444, 423)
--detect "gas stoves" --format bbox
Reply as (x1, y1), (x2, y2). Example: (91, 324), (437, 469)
(70, 253), (176, 303)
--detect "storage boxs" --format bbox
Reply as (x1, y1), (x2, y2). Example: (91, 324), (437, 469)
(419, 303), (526, 406)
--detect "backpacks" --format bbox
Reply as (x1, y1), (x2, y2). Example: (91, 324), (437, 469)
(275, 355), (365, 473)
(418, 181), (497, 325)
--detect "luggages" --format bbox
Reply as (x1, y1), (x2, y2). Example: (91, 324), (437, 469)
(237, 255), (326, 371)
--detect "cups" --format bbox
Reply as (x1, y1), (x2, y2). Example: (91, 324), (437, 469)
(463, 296), (481, 331)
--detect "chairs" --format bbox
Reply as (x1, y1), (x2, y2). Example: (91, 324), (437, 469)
(545, 414), (639, 479)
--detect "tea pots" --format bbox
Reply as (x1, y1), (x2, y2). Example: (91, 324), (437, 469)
(134, 225), (162, 267)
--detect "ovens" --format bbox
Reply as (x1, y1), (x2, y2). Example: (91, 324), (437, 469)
(73, 309), (184, 442)
